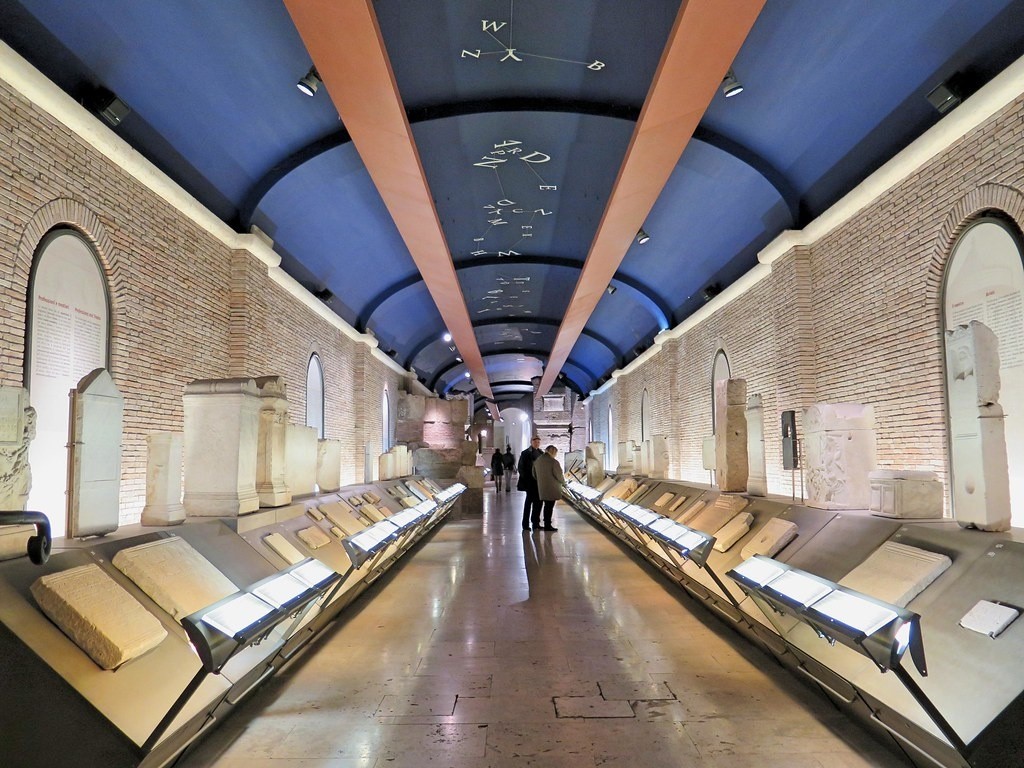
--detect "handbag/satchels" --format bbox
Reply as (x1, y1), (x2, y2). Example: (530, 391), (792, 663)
(512, 464), (517, 473)
(490, 474), (494, 481)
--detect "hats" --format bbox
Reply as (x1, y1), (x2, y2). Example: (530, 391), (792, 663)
(533, 435), (541, 440)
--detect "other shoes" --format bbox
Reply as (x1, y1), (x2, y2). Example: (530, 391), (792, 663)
(533, 522), (544, 529)
(506, 490), (510, 492)
(523, 526), (531, 531)
(545, 524), (558, 531)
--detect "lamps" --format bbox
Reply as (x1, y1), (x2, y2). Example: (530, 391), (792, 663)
(297, 63), (324, 96)
(634, 228), (650, 243)
(558, 373), (564, 380)
(606, 284), (617, 295)
(449, 345), (480, 395)
(719, 67), (744, 98)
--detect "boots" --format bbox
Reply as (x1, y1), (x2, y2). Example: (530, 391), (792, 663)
(495, 482), (498, 493)
(499, 482), (502, 491)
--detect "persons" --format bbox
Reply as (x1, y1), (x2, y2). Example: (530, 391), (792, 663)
(517, 437), (544, 531)
(532, 445), (564, 531)
(503, 447), (515, 492)
(491, 449), (503, 493)
